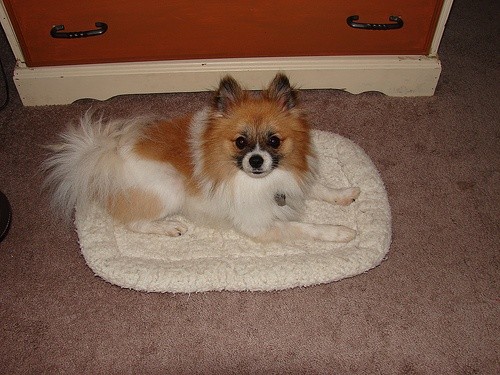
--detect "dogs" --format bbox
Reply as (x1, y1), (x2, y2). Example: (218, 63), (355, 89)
(32, 74), (360, 242)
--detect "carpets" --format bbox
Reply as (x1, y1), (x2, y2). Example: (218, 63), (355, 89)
(74, 129), (393, 294)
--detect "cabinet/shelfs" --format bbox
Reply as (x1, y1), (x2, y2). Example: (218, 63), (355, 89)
(0, 0), (453, 108)
